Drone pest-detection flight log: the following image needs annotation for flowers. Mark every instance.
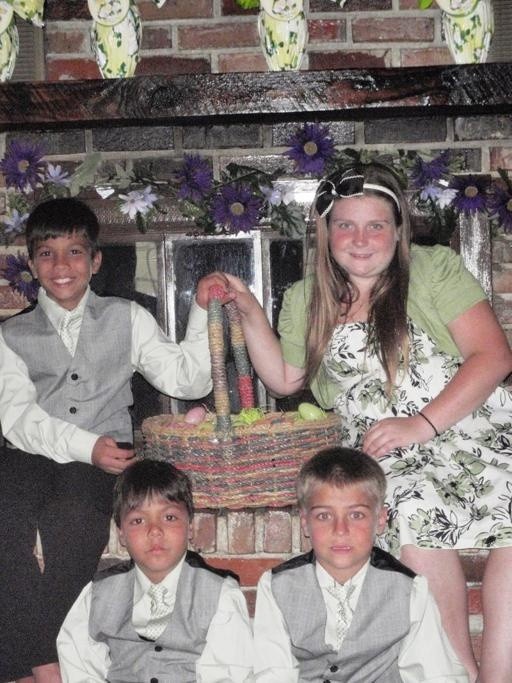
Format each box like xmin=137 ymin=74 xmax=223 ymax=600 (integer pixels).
xmin=0 ymin=120 xmax=512 ymax=303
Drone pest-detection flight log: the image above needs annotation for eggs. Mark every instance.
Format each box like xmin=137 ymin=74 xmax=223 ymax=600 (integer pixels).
xmin=298 ymin=401 xmax=324 ymax=421
xmin=183 ymin=405 xmax=207 ymax=427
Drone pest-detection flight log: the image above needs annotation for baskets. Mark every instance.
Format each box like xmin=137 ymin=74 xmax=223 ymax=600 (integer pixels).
xmin=140 ymin=284 xmax=343 ymax=513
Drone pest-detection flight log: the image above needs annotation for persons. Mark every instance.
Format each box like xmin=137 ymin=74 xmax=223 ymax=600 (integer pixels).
xmin=55 ymin=462 xmax=253 ymax=682
xmin=1 ymin=200 xmax=229 ymax=683
xmin=254 ymin=448 xmax=468 ymax=682
xmin=223 ymin=162 xmax=511 ymax=682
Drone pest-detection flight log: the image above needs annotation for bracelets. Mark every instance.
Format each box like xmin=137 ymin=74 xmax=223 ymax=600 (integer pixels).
xmin=418 ymin=412 xmax=439 ymax=436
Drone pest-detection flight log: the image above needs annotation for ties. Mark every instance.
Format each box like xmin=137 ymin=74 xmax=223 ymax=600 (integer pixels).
xmin=57 ymin=312 xmax=82 ymax=357
xmin=142 ymin=585 xmax=174 ymax=642
xmin=325 ymin=583 xmax=358 ymax=644
